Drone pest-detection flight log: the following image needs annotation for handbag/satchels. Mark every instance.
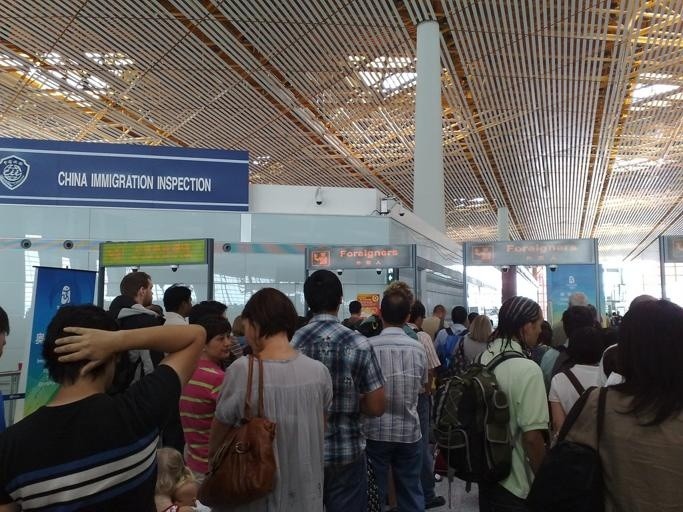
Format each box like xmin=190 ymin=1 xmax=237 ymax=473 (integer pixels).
xmin=524 ymin=386 xmax=609 ymax=511
xmin=197 ymin=353 xmax=279 ymax=511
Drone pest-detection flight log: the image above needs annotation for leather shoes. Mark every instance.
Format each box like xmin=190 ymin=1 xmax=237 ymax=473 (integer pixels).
xmin=426 ymin=495 xmax=446 ymax=508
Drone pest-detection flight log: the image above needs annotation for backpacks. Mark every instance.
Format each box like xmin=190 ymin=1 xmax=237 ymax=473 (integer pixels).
xmin=436 ymin=328 xmax=470 ymax=391
xmin=444 ymin=327 xmax=468 ymax=360
xmin=430 ymin=350 xmax=527 ymax=493
xmin=433 ymin=318 xmax=446 ymax=342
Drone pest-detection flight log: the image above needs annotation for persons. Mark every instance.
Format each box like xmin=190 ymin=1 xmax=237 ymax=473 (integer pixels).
xmin=405 ymin=299 xmax=445 ymax=509
xmin=420 ymin=305 xmax=447 ymax=344
xmin=178 ymin=314 xmax=232 ymax=484
xmin=340 ymin=301 xmax=367 ymax=331
xmin=526 ymin=321 xmax=552 ymax=366
xmin=205 ymin=287 xmax=333 ymax=512
xmin=467 ymin=313 xmax=478 ymax=325
xmin=0 ymin=303 xmax=206 ymax=512
xmin=188 ymin=300 xmax=227 ymax=325
xmin=360 ymin=289 xmax=428 ymax=512
xmin=171 ymin=479 xmax=211 ymax=512
xmin=231 ymin=315 xmax=248 ymax=350
xmin=154 ymin=448 xmax=193 ymax=512
xmin=469 ymin=296 xmax=548 ymax=512
xmin=548 ymin=320 xmax=607 ymax=435
xmin=288 ymin=269 xmax=386 ymax=512
xmin=596 ymin=344 xmax=625 ymax=387
xmin=550 ymin=292 xmax=587 ymax=348
xmin=539 ymin=305 xmax=606 ymax=399
xmin=450 ymin=315 xmax=493 ymax=365
xmin=161 ymin=283 xmax=192 ymax=359
xmin=104 ymin=272 xmax=165 ymax=393
xmin=433 ymin=306 xmax=471 ymax=351
xmin=0 ymin=307 xmax=9 ymax=431
xmin=382 ymin=280 xmax=418 ymax=341
xmin=550 ymin=299 xmax=682 ymax=512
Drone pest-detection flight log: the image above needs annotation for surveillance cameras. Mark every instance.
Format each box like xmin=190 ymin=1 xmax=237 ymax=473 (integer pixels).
xmin=131 ymin=266 xmax=140 ymax=272
xmin=502 ymin=265 xmax=509 ymax=272
xmin=550 ymin=264 xmax=557 ymax=271
xmin=397 ymin=206 xmax=404 ymax=216
xmin=171 ymin=265 xmax=179 ymax=271
xmin=375 ymin=268 xmax=381 ymax=274
xmin=337 ymin=269 xmax=343 ymax=275
xmin=316 ymin=196 xmax=322 ymax=204
